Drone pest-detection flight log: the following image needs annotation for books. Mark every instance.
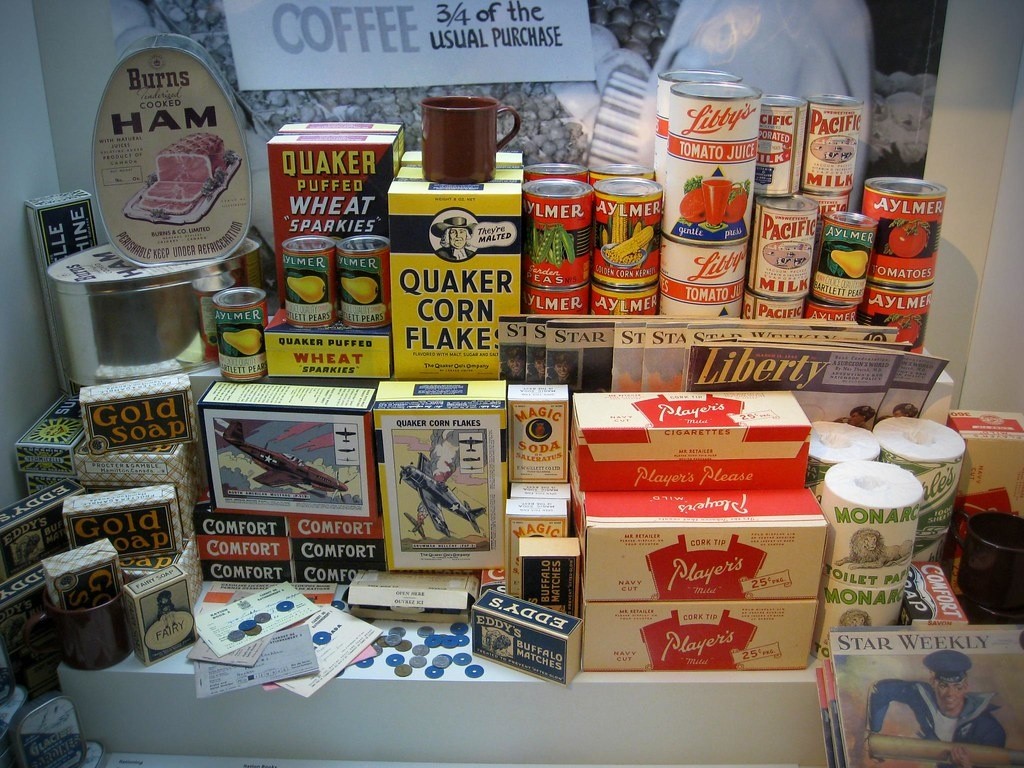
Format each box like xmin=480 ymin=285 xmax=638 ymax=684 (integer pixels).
xmin=499 ymin=315 xmax=949 ymax=429
xmin=814 ymin=625 xmax=1024 ymax=768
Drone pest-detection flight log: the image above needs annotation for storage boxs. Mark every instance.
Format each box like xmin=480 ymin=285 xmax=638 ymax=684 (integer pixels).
xmin=264 ymin=122 xmax=525 ymax=381
xmin=27 ymin=188 xmax=98 ymax=396
xmin=901 ymin=561 xmax=969 ymax=625
xmin=946 ymin=409 xmax=1024 ymax=598
xmin=571 ymin=391 xmax=831 ymax=672
xmin=50 ymin=34 xmax=260 ymax=388
xmin=0 ymin=374 xmax=203 ymax=767
xmin=193 ymin=373 xmax=583 ymax=686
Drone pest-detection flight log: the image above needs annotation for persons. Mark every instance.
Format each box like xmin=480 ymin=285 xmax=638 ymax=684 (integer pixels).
xmin=875 ymin=402 xmax=917 ymax=424
xmin=834 ymin=405 xmax=875 ymax=428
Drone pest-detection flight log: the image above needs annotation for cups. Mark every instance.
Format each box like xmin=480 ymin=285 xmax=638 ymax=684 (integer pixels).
xmin=950 ymin=508 xmax=1024 ymax=613
xmin=23 ymin=587 xmax=134 ymax=670
xmin=418 ymin=96 xmax=520 ymax=184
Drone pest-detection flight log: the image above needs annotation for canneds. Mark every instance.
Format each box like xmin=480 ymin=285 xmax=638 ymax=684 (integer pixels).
xmin=281 ymin=236 xmax=338 ymax=328
xmin=192 ymin=277 xmax=236 ymax=361
xmin=335 ymin=234 xmax=393 ymax=329
xmin=522 ymin=71 xmax=951 ymax=355
xmin=211 ymin=287 xmax=269 ymax=382
